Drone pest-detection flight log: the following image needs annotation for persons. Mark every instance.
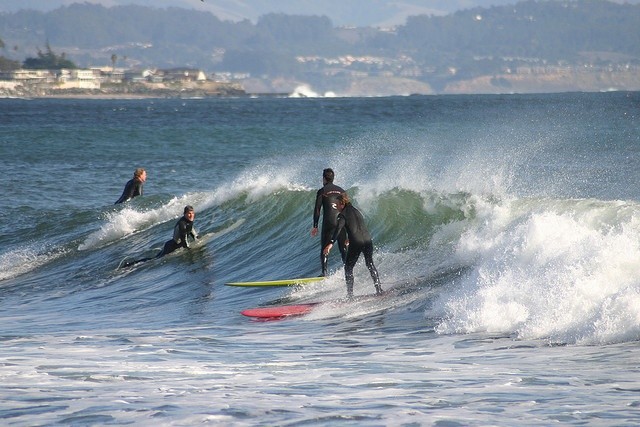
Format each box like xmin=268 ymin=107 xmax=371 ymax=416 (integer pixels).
xmin=310 ymin=168 xmax=348 ymax=277
xmin=323 ymin=193 xmax=383 ymax=299
xmin=123 ymin=205 xmax=197 ymax=268
xmin=114 ymin=168 xmax=146 ymax=205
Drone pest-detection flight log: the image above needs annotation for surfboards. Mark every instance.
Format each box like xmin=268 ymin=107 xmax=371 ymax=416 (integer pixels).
xmin=224 ymin=276 xmax=332 ymax=286
xmin=241 ymin=292 xmax=392 ymax=317
